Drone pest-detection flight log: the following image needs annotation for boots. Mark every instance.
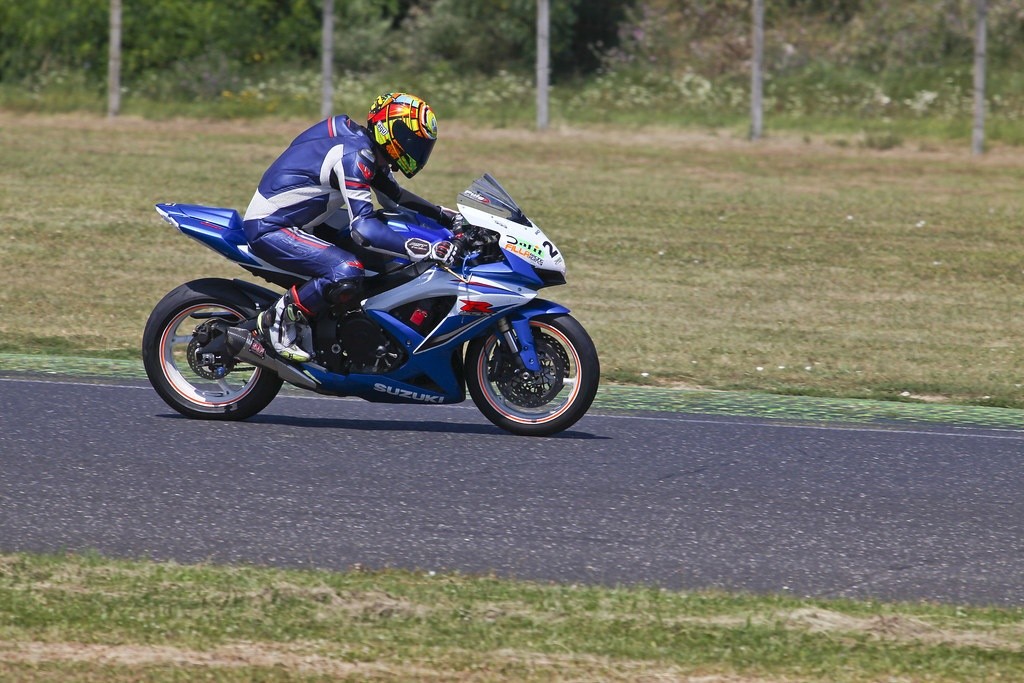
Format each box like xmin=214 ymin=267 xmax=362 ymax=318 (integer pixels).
xmin=256 ymin=283 xmax=318 ymax=363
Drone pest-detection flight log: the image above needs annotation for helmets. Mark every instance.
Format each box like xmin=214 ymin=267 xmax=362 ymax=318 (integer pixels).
xmin=368 ymin=93 xmax=438 ymax=179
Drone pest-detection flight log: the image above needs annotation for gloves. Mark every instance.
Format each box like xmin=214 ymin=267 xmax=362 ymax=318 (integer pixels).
xmin=405 ymin=236 xmax=460 ymax=267
xmin=437 ymin=206 xmax=470 ymax=235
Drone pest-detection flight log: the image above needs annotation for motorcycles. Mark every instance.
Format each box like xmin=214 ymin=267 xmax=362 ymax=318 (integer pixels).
xmin=142 ymin=172 xmax=601 ymax=437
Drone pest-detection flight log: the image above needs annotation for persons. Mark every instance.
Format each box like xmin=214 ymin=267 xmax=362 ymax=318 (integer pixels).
xmin=240 ymin=92 xmax=457 ymax=364
